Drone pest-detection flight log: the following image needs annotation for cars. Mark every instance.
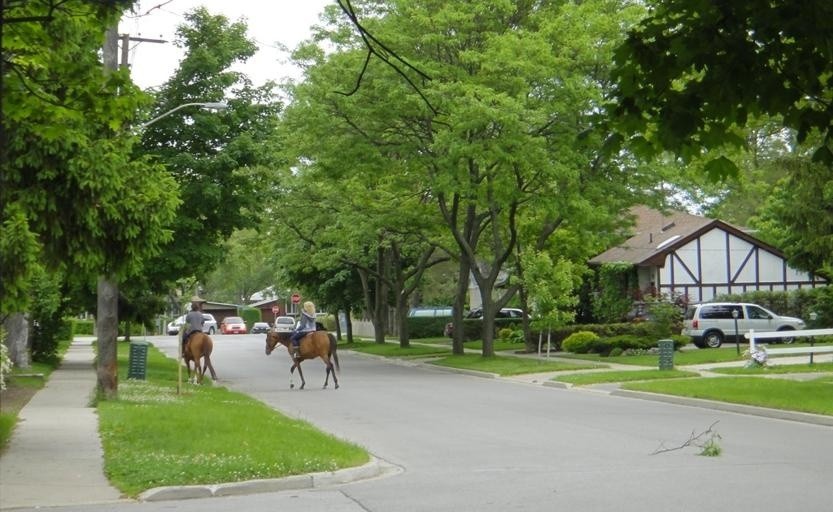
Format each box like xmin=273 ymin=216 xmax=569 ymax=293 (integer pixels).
xmin=167 ymin=313 xmax=296 ymax=335
xmin=445 ymin=307 xmax=533 ymax=342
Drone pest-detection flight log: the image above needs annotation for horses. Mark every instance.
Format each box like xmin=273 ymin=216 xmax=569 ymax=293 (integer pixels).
xmin=266 ymin=328 xmax=339 ymax=389
xmin=184 ymin=331 xmax=218 ymax=385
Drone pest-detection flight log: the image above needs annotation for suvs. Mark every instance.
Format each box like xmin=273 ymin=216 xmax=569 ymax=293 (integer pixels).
xmin=682 ymin=303 xmax=807 ymax=349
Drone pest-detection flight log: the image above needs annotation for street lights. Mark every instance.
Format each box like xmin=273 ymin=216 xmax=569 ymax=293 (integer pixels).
xmin=130 ymin=103 xmax=229 ymax=131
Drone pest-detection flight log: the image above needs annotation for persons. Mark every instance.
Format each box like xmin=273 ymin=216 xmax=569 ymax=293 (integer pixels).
xmin=289 ymin=300 xmax=316 ymax=359
xmin=181 ymin=303 xmax=204 ymax=358
